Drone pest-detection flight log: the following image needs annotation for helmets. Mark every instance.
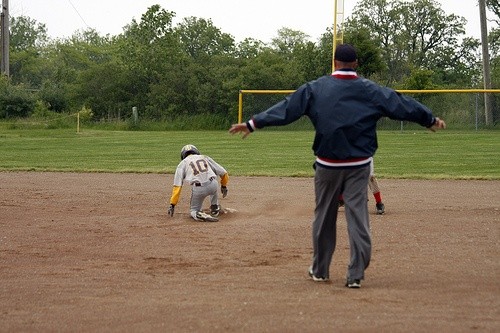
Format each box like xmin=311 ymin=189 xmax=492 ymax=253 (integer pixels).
xmin=181 ymin=144 xmax=200 ymax=159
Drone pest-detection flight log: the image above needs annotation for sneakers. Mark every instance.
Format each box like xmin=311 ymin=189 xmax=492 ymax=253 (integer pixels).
xmin=307 ymin=264 xmax=328 ymax=281
xmin=344 ymin=279 xmax=361 ymax=288
xmin=211 ymin=203 xmax=220 ymax=217
xmin=196 ymin=211 xmax=217 ymax=222
xmin=375 ymin=202 xmax=385 ymax=214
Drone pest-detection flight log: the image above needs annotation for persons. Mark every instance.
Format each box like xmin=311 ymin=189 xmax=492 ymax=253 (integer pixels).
xmin=229 ymin=45 xmax=446 ymax=289
xmin=368 ymin=170 xmax=385 ymax=214
xmin=167 ymin=144 xmax=229 ymax=222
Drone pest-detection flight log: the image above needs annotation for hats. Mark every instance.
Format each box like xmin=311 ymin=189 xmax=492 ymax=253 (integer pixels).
xmin=335 ymin=44 xmax=357 ymax=62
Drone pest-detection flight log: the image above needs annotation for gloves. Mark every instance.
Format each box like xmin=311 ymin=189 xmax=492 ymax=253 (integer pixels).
xmin=167 ymin=204 xmax=175 ymax=218
xmin=221 ymin=185 xmax=228 ymax=198
